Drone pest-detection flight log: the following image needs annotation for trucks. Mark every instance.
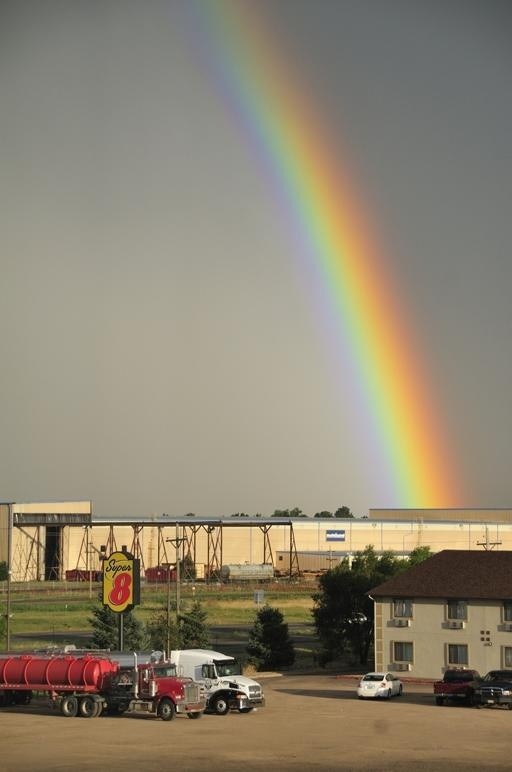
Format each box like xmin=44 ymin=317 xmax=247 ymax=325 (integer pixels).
xmin=142 ymin=566 xmax=182 ymax=583
xmin=64 ymin=568 xmax=103 ymax=582
xmin=26 ymin=643 xmax=265 ymax=715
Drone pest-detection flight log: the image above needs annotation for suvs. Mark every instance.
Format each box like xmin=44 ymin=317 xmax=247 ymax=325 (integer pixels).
xmin=474 ymin=670 xmax=512 ymax=711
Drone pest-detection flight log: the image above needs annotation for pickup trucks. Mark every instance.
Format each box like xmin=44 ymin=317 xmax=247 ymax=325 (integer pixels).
xmin=434 ymin=667 xmax=481 ymax=707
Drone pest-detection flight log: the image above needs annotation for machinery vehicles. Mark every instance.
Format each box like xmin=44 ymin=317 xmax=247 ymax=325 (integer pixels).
xmin=217 ymin=562 xmax=288 ymax=584
xmin=0 ymin=651 xmax=202 ymax=722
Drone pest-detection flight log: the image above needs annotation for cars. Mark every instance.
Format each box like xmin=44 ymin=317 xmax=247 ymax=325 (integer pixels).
xmin=343 ymin=610 xmax=368 ymax=623
xmin=351 ymin=671 xmax=406 ymax=700
